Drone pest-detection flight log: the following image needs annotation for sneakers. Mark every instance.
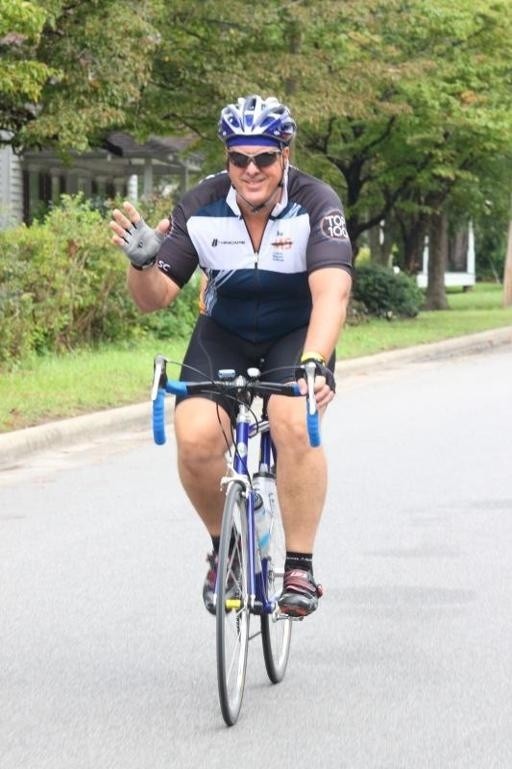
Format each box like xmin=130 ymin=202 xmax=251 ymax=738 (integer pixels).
xmin=202 ymin=548 xmax=241 ymax=617
xmin=277 ymin=564 xmax=325 ymax=618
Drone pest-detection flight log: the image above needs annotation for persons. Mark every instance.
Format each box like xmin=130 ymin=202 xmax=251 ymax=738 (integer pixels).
xmin=109 ymin=93 xmax=355 ymax=617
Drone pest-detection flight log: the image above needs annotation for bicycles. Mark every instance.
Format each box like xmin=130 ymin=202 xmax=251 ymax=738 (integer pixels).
xmin=142 ymin=354 xmax=333 ymax=730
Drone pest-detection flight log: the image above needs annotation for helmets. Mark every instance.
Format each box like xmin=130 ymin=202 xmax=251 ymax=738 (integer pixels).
xmin=215 ymin=93 xmax=297 ymax=146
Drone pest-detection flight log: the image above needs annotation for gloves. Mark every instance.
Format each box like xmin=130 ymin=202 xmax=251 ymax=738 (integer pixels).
xmin=116 ymin=215 xmax=167 ymax=270
xmin=294 ymin=358 xmax=337 ymax=394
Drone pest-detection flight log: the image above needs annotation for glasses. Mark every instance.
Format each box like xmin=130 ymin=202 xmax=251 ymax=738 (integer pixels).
xmin=225 ymin=148 xmax=283 ymax=169
